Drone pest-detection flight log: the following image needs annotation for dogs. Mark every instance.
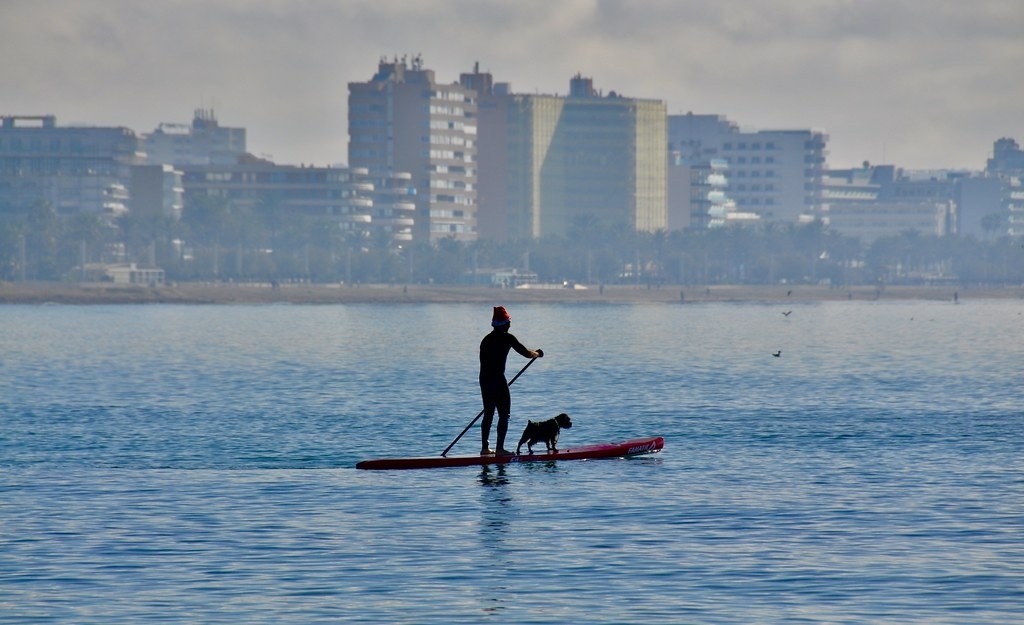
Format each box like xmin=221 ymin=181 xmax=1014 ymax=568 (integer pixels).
xmin=516 ymin=413 xmax=573 ymax=452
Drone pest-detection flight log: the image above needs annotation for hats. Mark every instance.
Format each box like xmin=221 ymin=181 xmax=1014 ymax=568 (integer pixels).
xmin=491 ymin=306 xmax=511 ymax=326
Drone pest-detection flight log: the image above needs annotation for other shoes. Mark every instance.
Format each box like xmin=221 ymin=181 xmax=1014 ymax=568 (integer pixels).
xmin=495 ymin=450 xmax=516 ymax=457
xmin=480 ymin=448 xmax=496 ymax=455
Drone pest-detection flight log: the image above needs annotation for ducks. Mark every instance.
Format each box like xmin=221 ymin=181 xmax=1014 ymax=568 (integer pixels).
xmin=772 ymin=351 xmax=781 ymax=358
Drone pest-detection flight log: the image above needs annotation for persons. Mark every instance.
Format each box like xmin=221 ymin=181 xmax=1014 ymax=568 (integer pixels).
xmin=479 ymin=305 xmax=544 ymax=455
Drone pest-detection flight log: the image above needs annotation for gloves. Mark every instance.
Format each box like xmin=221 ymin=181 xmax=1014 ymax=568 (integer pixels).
xmin=535 ymin=349 xmax=544 ymax=358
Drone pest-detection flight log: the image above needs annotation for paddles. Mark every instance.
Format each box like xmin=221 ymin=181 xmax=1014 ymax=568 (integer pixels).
xmin=439 ymin=356 xmax=537 ymax=457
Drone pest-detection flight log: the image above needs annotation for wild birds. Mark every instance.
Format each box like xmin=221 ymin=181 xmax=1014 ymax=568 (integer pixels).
xmin=782 ymin=311 xmax=793 ymax=315
